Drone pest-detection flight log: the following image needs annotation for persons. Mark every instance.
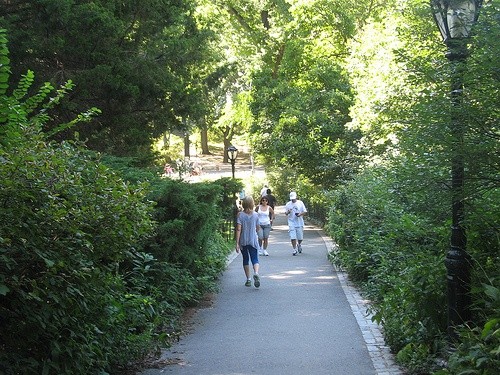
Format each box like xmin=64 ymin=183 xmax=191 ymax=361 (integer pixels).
xmin=239 ymin=188 xmax=245 ymax=208
xmin=235 ymin=197 xmax=261 ymax=288
xmin=285 ymin=192 xmax=307 ymax=255
xmin=254 ymin=184 xmax=276 ymax=256
xmin=164 ymin=159 xmax=203 ymax=177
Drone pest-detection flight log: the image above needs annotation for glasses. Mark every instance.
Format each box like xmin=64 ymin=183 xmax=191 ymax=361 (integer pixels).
xmin=262 ymin=200 xmax=267 ymax=202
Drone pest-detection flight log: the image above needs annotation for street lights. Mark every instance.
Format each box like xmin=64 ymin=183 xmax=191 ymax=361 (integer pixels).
xmin=226 ymin=145 xmax=239 ymax=227
xmin=430 ymin=1 xmax=488 ymax=344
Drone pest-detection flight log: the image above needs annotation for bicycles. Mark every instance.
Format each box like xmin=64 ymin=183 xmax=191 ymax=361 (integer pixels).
xmin=163 ymin=156 xmax=204 ymax=176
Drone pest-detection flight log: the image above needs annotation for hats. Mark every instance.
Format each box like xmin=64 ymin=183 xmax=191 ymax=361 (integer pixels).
xmin=290 ymin=192 xmax=296 ymax=199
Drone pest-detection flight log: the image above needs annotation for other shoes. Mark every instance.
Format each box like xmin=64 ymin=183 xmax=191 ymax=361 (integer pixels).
xmin=297 ymin=244 xmax=302 ymax=253
xmin=293 ymin=249 xmax=297 ymax=254
xmin=253 ymin=272 xmax=260 ymax=288
xmin=263 ymin=251 xmax=269 ymax=255
xmin=259 ymin=250 xmax=263 ymax=255
xmin=245 ymin=280 xmax=251 ymax=286
